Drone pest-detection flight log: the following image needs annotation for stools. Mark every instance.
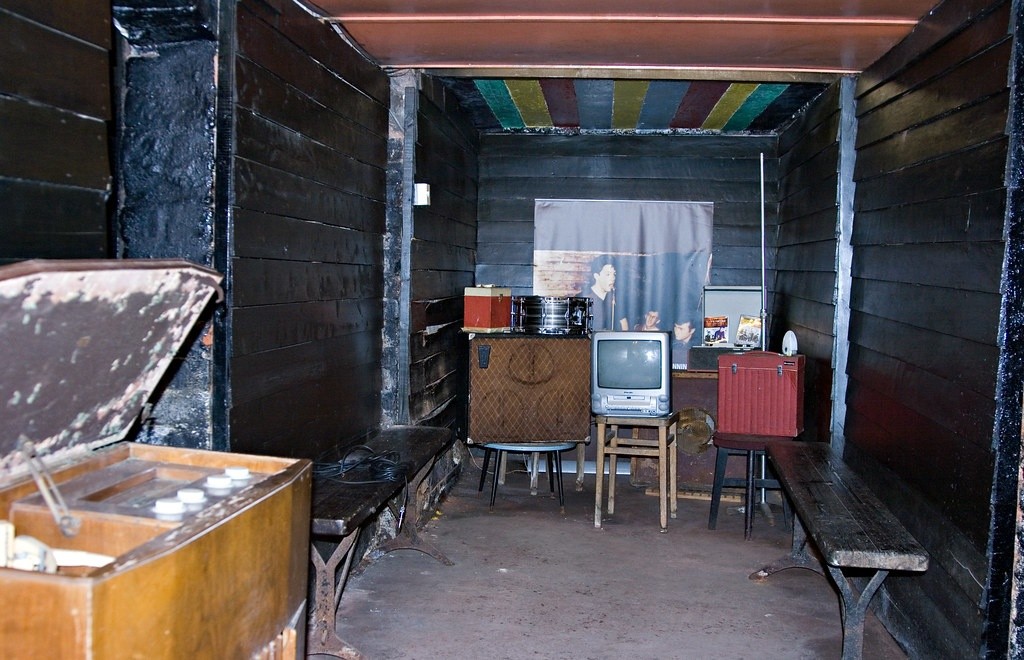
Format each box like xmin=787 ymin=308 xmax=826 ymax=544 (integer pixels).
xmin=708 ymin=435 xmax=790 ymax=540
xmin=594 ymin=412 xmax=679 ymax=530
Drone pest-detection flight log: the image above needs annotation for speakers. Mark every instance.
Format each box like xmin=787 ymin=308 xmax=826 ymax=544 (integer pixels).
xmin=467 ymin=332 xmax=590 ymax=445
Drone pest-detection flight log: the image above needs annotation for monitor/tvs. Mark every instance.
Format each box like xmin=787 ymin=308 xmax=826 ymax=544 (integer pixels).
xmin=591 ymin=330 xmax=671 ymax=418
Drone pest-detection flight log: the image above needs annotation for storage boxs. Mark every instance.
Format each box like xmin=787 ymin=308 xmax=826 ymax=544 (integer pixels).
xmin=711 ymin=354 xmax=804 ymax=436
xmin=461 ymin=287 xmax=514 ymax=334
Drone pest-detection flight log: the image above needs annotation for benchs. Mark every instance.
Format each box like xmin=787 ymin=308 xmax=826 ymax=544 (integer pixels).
xmin=748 ymin=435 xmax=932 ymax=659
xmin=304 ymin=423 xmax=462 ymax=660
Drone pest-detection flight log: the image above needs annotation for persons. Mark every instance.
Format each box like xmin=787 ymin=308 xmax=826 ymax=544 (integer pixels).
xmin=573 ymin=255 xmax=629 ymax=332
xmin=634 ymin=308 xmax=661 ymax=333
xmin=672 ymin=314 xmax=703 ymax=348
xmin=705 ymin=327 xmax=725 ymax=342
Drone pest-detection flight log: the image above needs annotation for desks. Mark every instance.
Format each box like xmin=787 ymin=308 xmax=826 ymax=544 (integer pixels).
xmin=476 ymin=440 xmax=576 ymax=511
